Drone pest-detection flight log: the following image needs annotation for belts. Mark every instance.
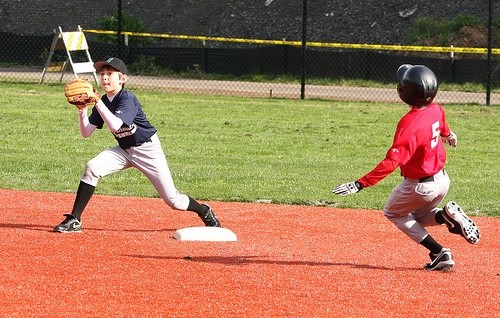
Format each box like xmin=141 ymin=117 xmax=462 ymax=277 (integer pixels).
xmin=419 ymin=168 xmax=445 ymax=183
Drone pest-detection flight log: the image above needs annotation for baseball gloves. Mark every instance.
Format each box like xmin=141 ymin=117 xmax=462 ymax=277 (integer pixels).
xmin=64 ymin=80 xmax=103 ymax=106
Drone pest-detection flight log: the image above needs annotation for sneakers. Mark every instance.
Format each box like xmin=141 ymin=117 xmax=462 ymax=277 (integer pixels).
xmin=53 ymin=213 xmax=82 ymax=232
xmin=442 ymin=201 xmax=480 ymax=244
xmin=198 ymin=204 xmax=221 ymax=228
xmin=423 ymin=248 xmax=455 ymax=270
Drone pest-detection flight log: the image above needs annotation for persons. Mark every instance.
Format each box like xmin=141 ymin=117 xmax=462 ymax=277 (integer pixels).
xmin=54 ymin=57 xmax=221 ymax=233
xmin=333 ymin=61 xmax=481 ymax=271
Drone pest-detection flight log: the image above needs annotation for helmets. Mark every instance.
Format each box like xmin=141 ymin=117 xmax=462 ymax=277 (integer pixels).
xmin=396 ymin=63 xmax=438 ymax=106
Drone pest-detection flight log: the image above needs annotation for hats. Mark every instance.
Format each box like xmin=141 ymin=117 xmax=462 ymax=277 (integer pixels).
xmin=94 ymin=57 xmax=128 ymax=75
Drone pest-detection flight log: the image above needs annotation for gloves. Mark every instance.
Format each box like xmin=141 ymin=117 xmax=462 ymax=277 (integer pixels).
xmin=332 ymin=180 xmax=363 ymax=196
xmin=441 ymin=132 xmax=458 ymax=147
